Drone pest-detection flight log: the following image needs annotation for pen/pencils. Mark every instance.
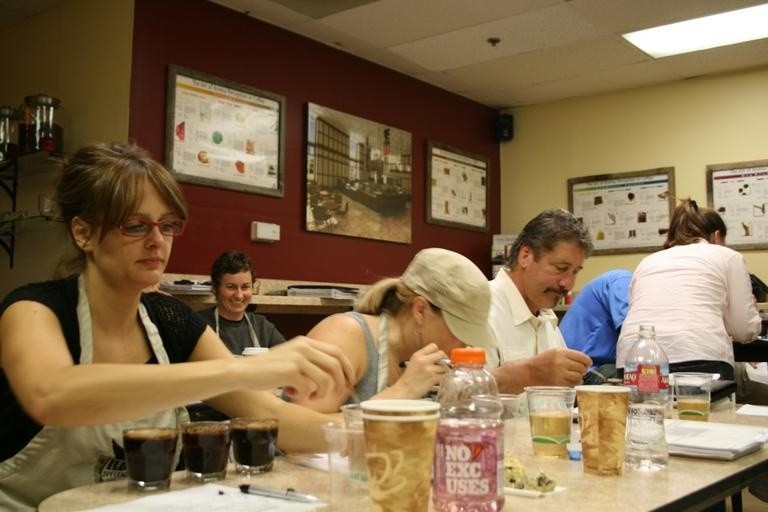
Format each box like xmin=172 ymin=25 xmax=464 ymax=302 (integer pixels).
xmin=399 ymin=359 xmax=452 ymax=367
xmin=238 ymin=484 xmax=317 ymax=503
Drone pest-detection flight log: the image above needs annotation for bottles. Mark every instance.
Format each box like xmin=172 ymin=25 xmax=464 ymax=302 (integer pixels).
xmin=625 ymin=324 xmax=669 ymax=473
xmin=431 ymin=347 xmax=506 ymax=511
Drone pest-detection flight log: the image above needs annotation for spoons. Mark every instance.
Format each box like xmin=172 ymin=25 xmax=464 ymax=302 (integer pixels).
xmin=588 ymin=367 xmax=623 ymax=383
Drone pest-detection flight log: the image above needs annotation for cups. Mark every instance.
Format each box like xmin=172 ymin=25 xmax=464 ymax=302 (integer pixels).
xmin=471 ymin=392 xmax=521 ymax=456
xmin=228 ymin=417 xmax=281 ymax=474
xmin=241 ymin=347 xmax=269 ymax=357
xmin=180 ymin=421 xmax=229 ymax=480
xmin=124 ymin=427 xmax=176 ymax=490
xmin=672 ymin=371 xmax=714 ymax=422
xmin=321 ymin=397 xmax=442 ymax=511
xmin=575 ymin=384 xmax=631 ymax=476
xmin=523 ymin=385 xmax=577 ymax=458
xmin=564 ymin=290 xmax=571 ymax=304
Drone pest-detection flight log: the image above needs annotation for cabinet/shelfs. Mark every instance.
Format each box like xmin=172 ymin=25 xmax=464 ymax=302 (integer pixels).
xmin=1 ymin=145 xmax=68 ymax=269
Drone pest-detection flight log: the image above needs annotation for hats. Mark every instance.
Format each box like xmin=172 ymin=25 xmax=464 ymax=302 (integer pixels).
xmin=400 ymin=248 xmax=498 ymax=348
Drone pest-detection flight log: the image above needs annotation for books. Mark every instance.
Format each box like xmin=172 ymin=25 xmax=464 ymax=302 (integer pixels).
xmin=667 ymin=418 xmax=761 ymax=460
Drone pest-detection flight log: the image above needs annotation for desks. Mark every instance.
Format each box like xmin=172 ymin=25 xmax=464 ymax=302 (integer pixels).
xmin=34 ymin=400 xmax=768 ymax=511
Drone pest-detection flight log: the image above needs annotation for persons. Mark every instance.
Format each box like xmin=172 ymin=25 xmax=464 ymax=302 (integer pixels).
xmin=557 ymin=269 xmax=633 ymax=384
xmin=0 ymin=141 xmax=356 ymax=512
xmin=195 ymin=250 xmax=285 ymax=354
xmin=280 ymin=248 xmax=494 ymax=413
xmin=483 ymin=208 xmax=593 ymax=394
xmin=615 ymin=198 xmax=761 ymax=380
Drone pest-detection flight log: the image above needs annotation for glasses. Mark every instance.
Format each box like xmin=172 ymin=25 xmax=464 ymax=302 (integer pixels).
xmin=87 ymin=214 xmax=187 ymax=237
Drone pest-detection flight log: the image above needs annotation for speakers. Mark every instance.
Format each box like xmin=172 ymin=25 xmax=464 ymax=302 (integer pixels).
xmin=495 ymin=115 xmax=513 ymax=140
